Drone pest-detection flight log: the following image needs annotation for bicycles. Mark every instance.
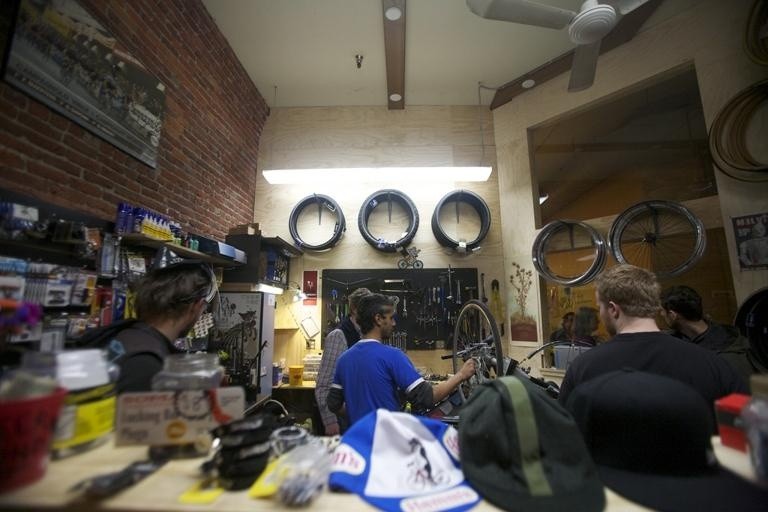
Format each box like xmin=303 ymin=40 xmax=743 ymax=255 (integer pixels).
xmin=443 ymin=299 xmax=597 ymax=404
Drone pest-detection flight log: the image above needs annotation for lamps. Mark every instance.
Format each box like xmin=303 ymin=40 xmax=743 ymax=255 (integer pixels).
xmin=260 ymin=160 xmax=491 ymax=188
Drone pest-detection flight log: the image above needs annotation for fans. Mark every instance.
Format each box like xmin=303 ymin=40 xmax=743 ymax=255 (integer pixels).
xmin=466 ymin=0 xmax=656 ymax=93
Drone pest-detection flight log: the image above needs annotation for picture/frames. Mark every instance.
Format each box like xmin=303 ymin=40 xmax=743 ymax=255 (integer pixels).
xmin=503 ymin=253 xmax=544 ymax=348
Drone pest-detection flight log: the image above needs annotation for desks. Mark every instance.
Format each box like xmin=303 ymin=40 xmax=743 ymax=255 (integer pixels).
xmin=274 ymin=374 xmax=317 ymax=394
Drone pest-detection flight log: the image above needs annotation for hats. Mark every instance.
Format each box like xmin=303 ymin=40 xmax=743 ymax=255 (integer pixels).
xmin=561 ymin=312 xmax=574 ymax=321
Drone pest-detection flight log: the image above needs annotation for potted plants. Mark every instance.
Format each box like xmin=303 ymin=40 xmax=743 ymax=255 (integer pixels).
xmin=507 ymin=259 xmax=540 ymax=344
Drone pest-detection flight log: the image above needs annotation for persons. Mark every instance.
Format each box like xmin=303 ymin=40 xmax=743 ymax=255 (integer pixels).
xmin=558 ymin=263 xmax=747 ymax=418
xmin=312 ymin=287 xmax=376 ymax=432
xmin=660 ymin=284 xmax=752 ymax=397
xmin=329 ymin=292 xmax=476 ymax=426
xmin=90 ymin=264 xmax=216 ymax=391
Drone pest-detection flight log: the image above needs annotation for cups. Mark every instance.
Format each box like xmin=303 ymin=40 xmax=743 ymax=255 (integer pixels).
xmin=270 ymin=425 xmax=308 ymax=456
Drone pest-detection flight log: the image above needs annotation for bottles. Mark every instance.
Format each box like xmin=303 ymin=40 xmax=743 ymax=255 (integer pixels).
xmin=741 ymin=373 xmax=768 ymax=493
xmin=148 ymin=353 xmax=223 ymax=461
xmin=288 ymin=366 xmax=304 ymax=386
xmin=273 ymin=362 xmax=282 ymax=385
